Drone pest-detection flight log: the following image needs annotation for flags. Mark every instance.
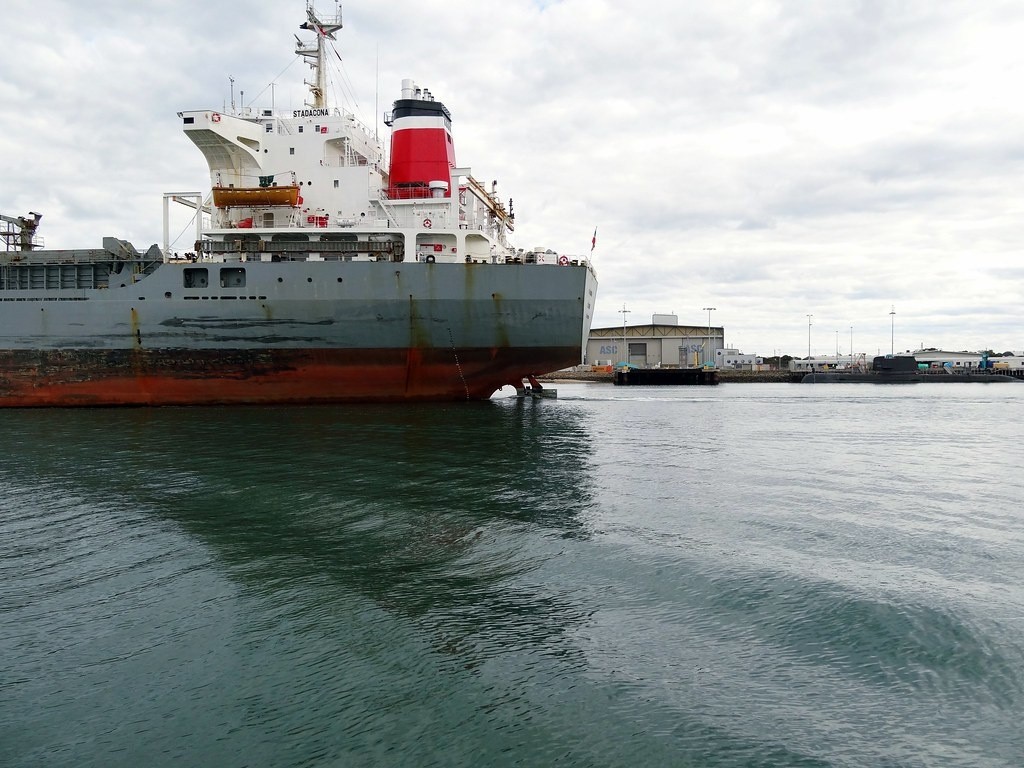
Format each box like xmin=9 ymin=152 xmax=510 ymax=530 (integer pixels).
xmin=592 ymin=229 xmax=597 ymax=249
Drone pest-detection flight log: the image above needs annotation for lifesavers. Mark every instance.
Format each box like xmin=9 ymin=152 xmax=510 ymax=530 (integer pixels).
xmin=423 ymin=217 xmax=432 ymax=229
xmin=560 ymin=255 xmax=569 ymax=265
xmin=425 ymin=254 xmax=436 ymax=265
xmin=211 ymin=112 xmax=220 ymax=122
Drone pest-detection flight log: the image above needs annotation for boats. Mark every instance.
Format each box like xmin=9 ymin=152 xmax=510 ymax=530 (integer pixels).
xmin=0 ymin=0 xmax=597 ymax=407
xmin=612 ymin=367 xmax=720 ymax=384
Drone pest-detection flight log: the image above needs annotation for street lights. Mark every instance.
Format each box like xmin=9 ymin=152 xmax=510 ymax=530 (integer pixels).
xmin=850 ymin=327 xmax=853 ymax=374
xmin=889 ymin=312 xmax=896 ymax=357
xmin=806 ymin=315 xmax=813 ymax=370
xmin=618 ymin=311 xmax=631 ymax=366
xmin=703 ymin=308 xmax=717 ymax=362
